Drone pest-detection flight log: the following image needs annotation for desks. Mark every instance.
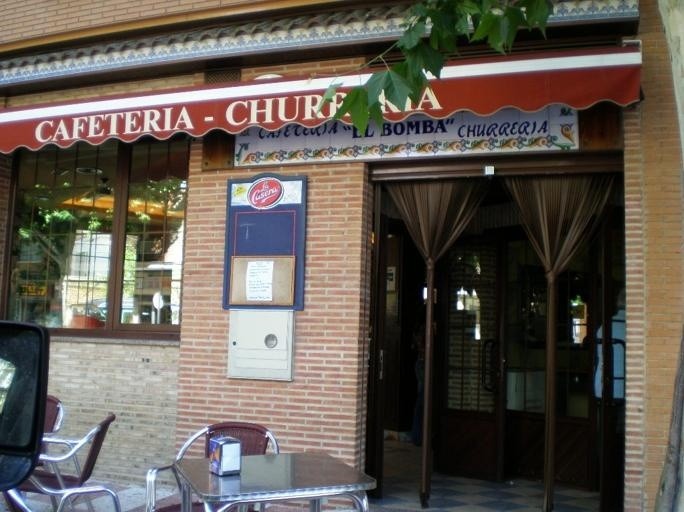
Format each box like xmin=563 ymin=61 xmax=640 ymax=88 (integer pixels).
xmin=174 ymin=452 xmax=377 ymax=512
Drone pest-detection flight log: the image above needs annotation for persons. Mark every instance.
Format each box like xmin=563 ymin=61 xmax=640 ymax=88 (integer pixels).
xmin=590 ymin=287 xmax=624 ymax=511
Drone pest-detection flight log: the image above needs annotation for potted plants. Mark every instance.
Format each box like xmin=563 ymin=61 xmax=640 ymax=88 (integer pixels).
xmin=71 ymin=308 xmax=105 ymax=328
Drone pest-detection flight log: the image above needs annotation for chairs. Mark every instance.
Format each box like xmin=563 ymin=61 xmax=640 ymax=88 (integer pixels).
xmin=3 ymin=395 xmax=120 ymax=512
xmin=146 ymin=423 xmax=279 ymax=512
